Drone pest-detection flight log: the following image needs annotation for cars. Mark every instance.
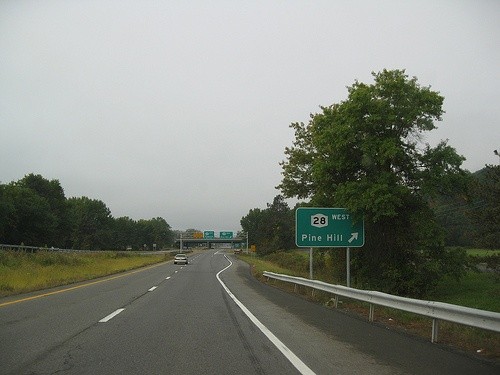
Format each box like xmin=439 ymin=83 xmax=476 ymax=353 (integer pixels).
xmin=174 ymin=253 xmax=188 ymax=264
